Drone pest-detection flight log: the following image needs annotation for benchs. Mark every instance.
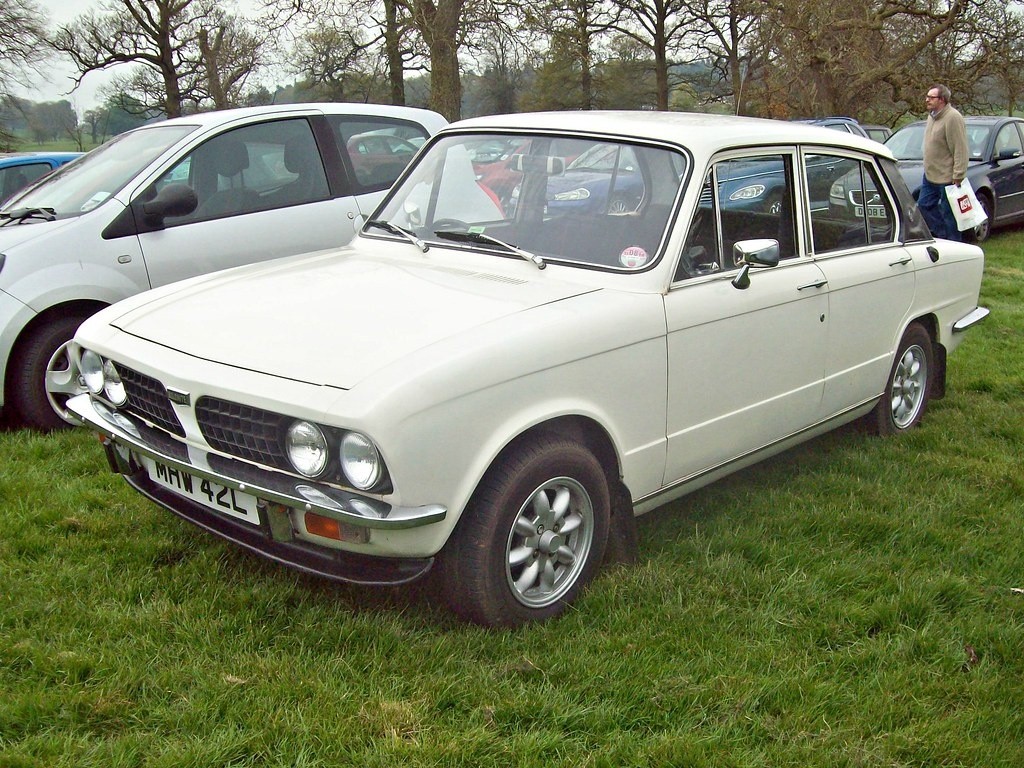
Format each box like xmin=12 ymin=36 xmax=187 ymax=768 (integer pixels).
xmin=693 ymin=207 xmax=847 ymax=266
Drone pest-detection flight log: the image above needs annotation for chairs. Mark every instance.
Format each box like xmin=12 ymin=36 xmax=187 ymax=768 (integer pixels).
xmin=194 ymin=142 xmax=258 ymax=218
xmin=277 ymin=136 xmax=327 ymax=202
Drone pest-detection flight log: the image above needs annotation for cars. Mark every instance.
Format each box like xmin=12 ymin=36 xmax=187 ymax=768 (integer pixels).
xmin=0 ymin=102 xmax=508 ymax=439
xmin=0 ymin=149 xmax=92 ymax=210
xmin=829 ymin=114 xmax=1024 ymax=243
xmin=159 ymin=115 xmax=896 ymax=219
xmin=61 ymin=108 xmax=992 ymax=633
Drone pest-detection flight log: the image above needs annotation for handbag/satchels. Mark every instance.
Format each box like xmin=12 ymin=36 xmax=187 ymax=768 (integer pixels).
xmin=944 ymin=177 xmax=988 ymax=231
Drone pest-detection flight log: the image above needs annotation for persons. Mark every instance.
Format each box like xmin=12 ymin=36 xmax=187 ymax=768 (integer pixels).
xmin=917 ymin=83 xmax=969 ymax=241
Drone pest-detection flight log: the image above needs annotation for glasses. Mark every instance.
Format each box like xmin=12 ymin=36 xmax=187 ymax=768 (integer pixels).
xmin=926 ymin=95 xmax=940 ymax=101
